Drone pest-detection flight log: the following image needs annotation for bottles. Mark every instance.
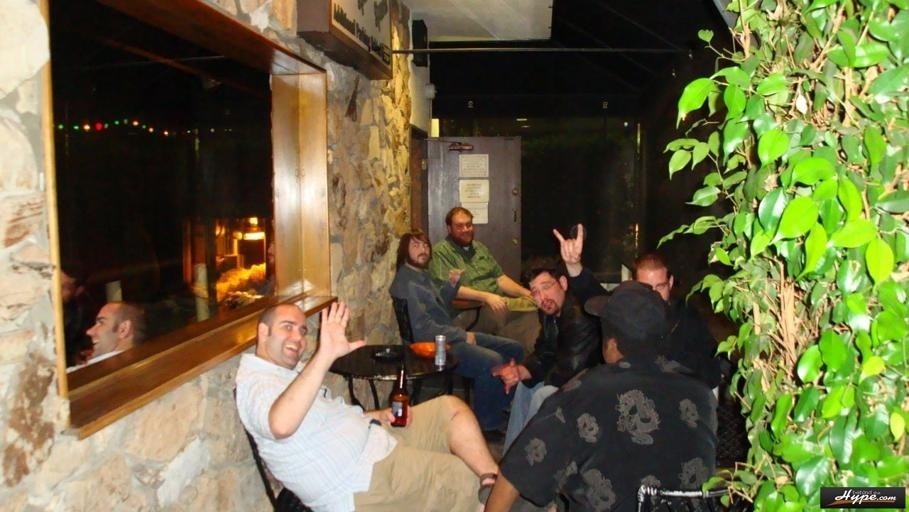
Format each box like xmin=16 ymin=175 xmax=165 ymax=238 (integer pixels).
xmin=434 ymin=334 xmax=446 ymax=369
xmin=387 ymin=363 xmax=408 ymax=428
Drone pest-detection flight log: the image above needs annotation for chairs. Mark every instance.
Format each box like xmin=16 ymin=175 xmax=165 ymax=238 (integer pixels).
xmin=226 ymin=393 xmax=314 ymax=511
xmin=393 ymin=298 xmax=414 ymax=342
xmin=636 ymin=484 xmax=758 ymax=512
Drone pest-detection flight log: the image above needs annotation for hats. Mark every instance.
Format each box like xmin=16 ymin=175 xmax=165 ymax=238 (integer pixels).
xmin=583 ymin=280 xmax=669 ymax=343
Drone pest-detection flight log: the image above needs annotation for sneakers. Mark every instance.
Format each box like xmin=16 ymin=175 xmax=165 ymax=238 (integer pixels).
xmin=482 ymin=409 xmax=512 ymax=445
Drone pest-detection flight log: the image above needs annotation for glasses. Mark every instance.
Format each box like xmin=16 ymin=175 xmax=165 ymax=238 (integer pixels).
xmin=529 ymin=280 xmax=559 ymax=298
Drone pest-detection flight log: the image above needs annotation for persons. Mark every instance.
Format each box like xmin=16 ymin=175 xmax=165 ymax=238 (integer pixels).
xmin=86 ymin=301 xmax=149 ymax=368
xmin=423 ymin=207 xmax=533 ymax=336
xmin=483 ymin=278 xmax=720 ymax=512
xmin=61 ymin=262 xmax=89 ymax=308
xmin=387 ymin=229 xmax=526 ymax=445
xmin=551 ymin=222 xmax=720 ymax=393
xmin=233 ymin=301 xmax=546 ymax=512
xmin=491 ymin=265 xmax=604 ymax=460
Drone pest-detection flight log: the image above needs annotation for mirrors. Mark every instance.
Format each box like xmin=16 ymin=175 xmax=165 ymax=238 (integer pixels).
xmin=40 ymin=0 xmax=340 ymax=438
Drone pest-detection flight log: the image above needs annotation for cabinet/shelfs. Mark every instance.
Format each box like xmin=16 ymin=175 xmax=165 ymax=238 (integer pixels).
xmin=452 ymin=298 xmax=487 ymax=335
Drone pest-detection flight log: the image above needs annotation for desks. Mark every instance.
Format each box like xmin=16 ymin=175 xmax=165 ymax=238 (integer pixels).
xmin=332 ymin=342 xmax=456 ymax=409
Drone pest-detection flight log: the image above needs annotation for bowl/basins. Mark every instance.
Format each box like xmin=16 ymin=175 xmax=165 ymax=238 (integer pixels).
xmin=369 ymin=348 xmax=407 ymax=363
xmin=409 ymin=341 xmax=452 ymax=359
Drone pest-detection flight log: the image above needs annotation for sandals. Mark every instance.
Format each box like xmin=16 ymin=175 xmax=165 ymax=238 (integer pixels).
xmin=478 ymin=471 xmax=561 ymax=512
